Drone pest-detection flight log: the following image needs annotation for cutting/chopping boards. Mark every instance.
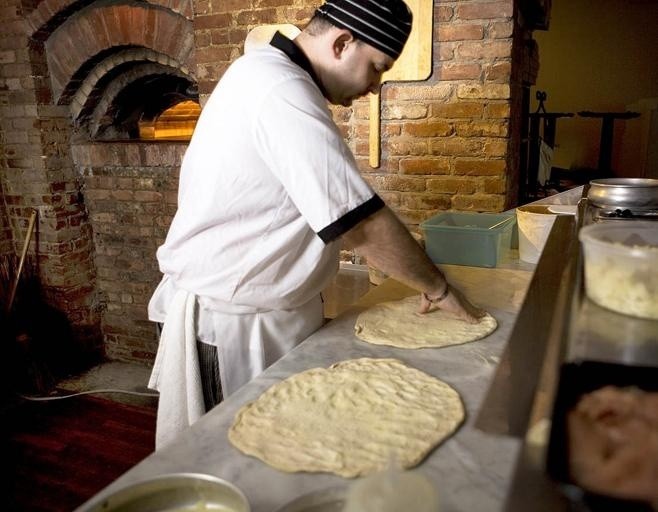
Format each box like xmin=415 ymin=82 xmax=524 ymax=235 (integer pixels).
xmin=367 ymin=1 xmax=433 ymax=169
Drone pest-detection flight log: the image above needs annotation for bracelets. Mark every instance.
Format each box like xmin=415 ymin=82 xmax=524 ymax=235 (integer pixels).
xmin=424 ymin=279 xmax=449 ymax=304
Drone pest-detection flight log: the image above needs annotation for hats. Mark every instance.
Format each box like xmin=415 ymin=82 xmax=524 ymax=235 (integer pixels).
xmin=315 ymin=0 xmax=413 ymax=60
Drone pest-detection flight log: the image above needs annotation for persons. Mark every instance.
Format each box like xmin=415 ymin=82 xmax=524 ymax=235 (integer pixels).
xmin=148 ymin=1 xmax=486 ymax=453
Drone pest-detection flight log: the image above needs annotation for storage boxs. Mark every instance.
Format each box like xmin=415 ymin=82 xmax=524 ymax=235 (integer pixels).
xmin=420 ymin=210 xmax=518 ymax=267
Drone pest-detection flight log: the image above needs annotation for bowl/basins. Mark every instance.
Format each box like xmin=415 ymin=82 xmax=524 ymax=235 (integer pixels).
xmin=577 ymin=219 xmax=658 ymax=319
xmin=586 ymin=178 xmax=658 ymax=211
xmin=82 ymin=473 xmax=249 ymax=512
